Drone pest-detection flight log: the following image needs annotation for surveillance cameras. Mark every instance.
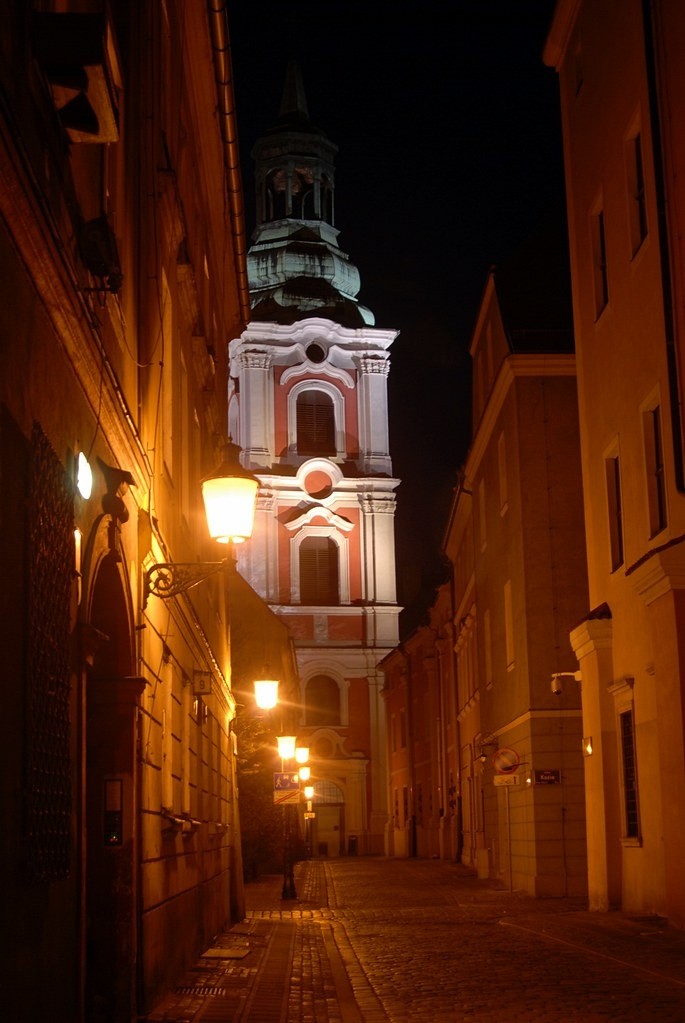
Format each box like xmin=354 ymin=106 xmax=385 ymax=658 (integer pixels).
xmin=551 ymin=679 xmax=562 ymax=695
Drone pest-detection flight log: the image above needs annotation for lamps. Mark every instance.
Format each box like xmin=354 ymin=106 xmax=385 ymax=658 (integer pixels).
xmin=229 ymin=666 xmax=279 ymax=736
xmin=143 ymin=433 xmax=261 ymax=607
xmin=480 ymin=743 xmax=497 ymax=762
